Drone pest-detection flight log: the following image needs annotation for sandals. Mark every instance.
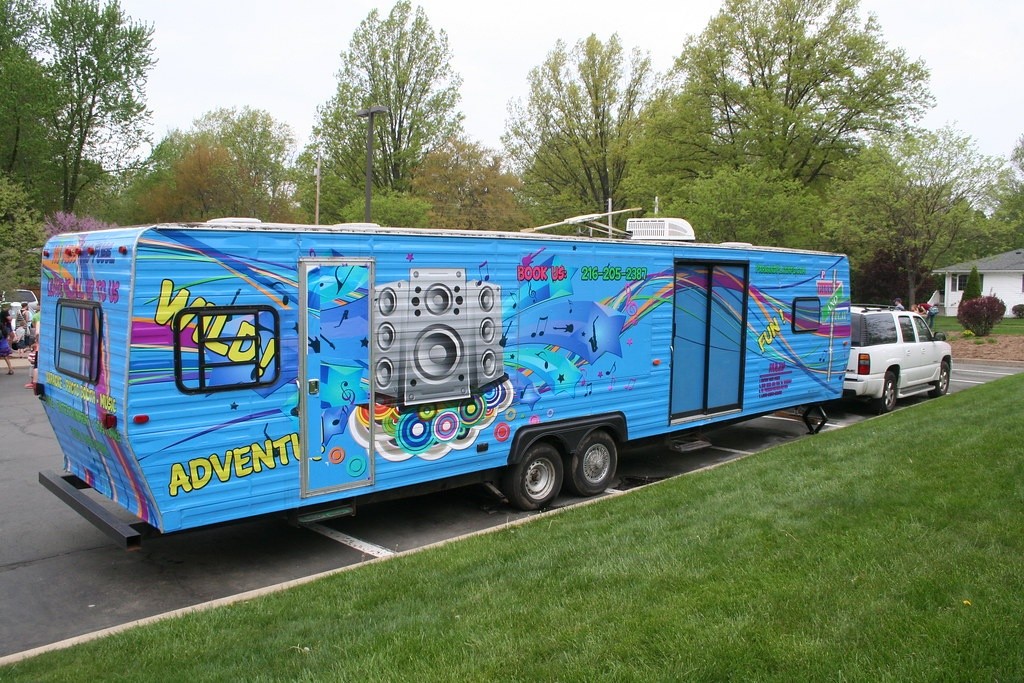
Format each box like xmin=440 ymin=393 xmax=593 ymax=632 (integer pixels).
xmin=7 ymin=368 xmax=13 ymax=374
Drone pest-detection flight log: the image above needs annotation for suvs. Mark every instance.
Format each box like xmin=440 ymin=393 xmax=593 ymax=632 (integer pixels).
xmin=839 ymin=303 xmax=954 ymax=414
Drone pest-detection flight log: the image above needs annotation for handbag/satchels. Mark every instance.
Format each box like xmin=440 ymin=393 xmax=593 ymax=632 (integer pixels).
xmin=926 ymin=306 xmax=939 ymax=315
xmin=26 ymin=324 xmax=29 ymax=336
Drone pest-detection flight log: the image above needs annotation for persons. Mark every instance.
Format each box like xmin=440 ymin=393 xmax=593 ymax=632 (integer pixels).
xmin=893 ymin=298 xmax=905 ymax=311
xmin=15 ymin=302 xmax=30 ymax=352
xmin=25 ymin=343 xmax=37 ymax=389
xmin=0 ymin=303 xmax=15 ymax=349
xmin=0 ymin=317 xmax=14 ymax=375
xmin=911 ymin=304 xmax=919 ymax=316
xmin=15 ymin=321 xmax=26 ymax=358
xmin=29 ymin=303 xmax=40 ymax=350
xmin=917 ymin=303 xmax=936 ymax=329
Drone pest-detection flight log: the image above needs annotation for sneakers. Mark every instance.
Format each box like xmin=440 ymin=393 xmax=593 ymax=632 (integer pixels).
xmin=24 ymin=382 xmax=34 ymax=388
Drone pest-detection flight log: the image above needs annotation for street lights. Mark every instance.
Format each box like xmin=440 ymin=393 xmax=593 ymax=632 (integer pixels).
xmin=356 ymin=106 xmax=388 ymax=223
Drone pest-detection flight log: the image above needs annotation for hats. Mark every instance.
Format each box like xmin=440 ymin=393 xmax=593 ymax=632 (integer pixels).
xmin=893 ymin=296 xmax=902 ymax=302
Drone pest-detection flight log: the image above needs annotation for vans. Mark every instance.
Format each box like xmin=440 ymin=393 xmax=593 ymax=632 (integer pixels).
xmin=0 ymin=289 xmax=39 ymax=331
